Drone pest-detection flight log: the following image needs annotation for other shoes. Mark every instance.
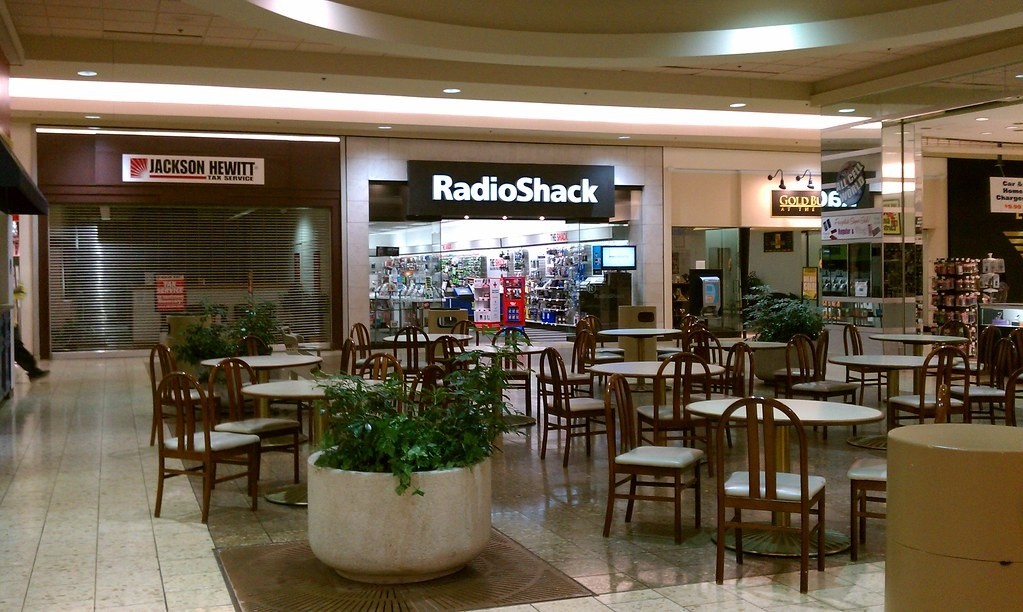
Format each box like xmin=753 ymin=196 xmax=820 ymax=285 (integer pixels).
xmin=26 ymin=368 xmax=50 ymax=382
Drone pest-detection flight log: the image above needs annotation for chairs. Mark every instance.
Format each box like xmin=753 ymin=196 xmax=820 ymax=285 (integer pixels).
xmin=149 ymin=313 xmax=1023 ymax=594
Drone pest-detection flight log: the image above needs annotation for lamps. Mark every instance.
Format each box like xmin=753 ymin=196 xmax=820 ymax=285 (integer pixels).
xmin=768 ymin=169 xmax=786 ymax=189
xmin=796 ymin=169 xmax=815 ymax=189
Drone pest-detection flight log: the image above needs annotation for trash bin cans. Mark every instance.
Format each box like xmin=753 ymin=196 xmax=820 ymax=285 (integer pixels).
xmin=884 ymin=424 xmax=1023 ymax=611
xmin=167 ymin=311 xmax=212 ymax=388
xmin=425 ymin=307 xmax=469 ymax=370
xmin=618 ymin=305 xmax=657 ymax=362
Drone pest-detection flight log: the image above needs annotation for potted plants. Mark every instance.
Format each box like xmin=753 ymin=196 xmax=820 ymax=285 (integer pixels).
xmin=741 ymin=285 xmax=827 ymax=386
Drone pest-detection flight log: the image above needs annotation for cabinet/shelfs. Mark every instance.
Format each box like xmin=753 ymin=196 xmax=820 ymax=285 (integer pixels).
xmin=821 ymin=243 xmax=1005 ymax=358
xmin=369 ymin=245 xmax=633 ymax=343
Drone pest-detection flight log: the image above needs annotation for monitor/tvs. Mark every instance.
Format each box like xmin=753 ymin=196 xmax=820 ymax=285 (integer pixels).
xmin=601 ymin=246 xmax=637 ymax=270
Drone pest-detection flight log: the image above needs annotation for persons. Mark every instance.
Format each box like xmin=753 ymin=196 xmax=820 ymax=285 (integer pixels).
xmin=12 ymin=327 xmax=51 ymax=384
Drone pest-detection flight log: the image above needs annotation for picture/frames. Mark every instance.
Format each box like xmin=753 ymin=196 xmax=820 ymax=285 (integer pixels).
xmin=882 ymin=198 xmax=902 ymax=236
xmin=763 ymin=230 xmax=794 ymax=253
